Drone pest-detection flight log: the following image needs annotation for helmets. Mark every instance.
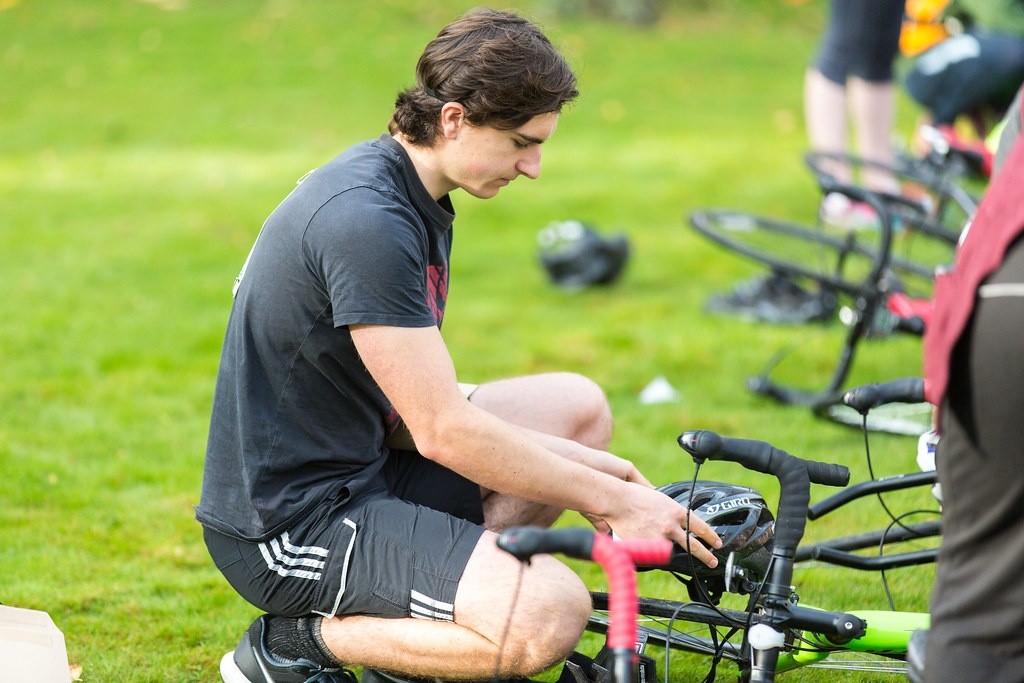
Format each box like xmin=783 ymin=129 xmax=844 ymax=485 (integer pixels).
xmin=648 ymin=479 xmax=775 ymax=602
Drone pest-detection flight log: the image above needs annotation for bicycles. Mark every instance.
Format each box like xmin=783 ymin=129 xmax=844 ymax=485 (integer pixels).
xmin=366 ymin=148 xmax=981 ymax=683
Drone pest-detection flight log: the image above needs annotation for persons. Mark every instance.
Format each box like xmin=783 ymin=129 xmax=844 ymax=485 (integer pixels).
xmin=802 ymin=0 xmax=1024 ymax=683
xmin=194 ymin=9 xmax=723 ymax=683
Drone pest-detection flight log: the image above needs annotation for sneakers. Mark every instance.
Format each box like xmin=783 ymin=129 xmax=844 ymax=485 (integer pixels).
xmin=361 ymin=667 xmax=422 ymax=683
xmin=220 ymin=614 xmax=357 ymax=683
